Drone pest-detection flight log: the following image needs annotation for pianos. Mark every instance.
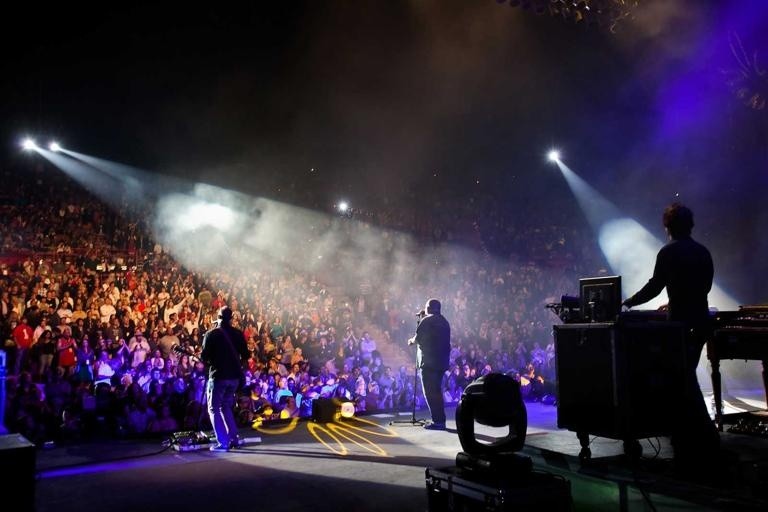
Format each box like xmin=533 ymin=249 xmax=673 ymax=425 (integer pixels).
xmin=557 ymin=306 xmax=740 ymax=325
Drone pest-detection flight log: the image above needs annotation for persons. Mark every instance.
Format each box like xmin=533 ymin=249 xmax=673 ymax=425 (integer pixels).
xmin=231 ymin=158 xmax=616 ymax=421
xmin=622 ymin=201 xmax=740 ymax=478
xmin=408 ymin=299 xmax=451 ymax=430
xmin=1 ymin=160 xmax=231 ymax=441
xmin=200 ymin=306 xmax=249 ymax=450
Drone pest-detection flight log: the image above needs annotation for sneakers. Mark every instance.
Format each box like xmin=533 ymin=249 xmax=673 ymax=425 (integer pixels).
xmin=423 ymin=423 xmax=445 ymax=430
xmin=210 ymin=433 xmax=244 ymax=451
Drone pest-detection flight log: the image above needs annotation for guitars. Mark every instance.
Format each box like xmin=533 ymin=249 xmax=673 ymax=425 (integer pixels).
xmin=172 ymin=345 xmax=255 ymax=394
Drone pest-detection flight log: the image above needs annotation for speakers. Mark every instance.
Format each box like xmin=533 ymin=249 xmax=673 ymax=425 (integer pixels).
xmin=312 ymin=397 xmax=355 ymax=423
xmin=0 ymin=432 xmax=37 ymax=512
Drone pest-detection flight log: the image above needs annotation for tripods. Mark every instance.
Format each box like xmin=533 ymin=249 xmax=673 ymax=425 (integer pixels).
xmin=390 ymin=317 xmax=426 ymax=427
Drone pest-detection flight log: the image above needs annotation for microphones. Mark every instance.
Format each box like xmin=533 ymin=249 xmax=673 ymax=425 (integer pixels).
xmin=418 ymin=311 xmax=425 ymax=317
